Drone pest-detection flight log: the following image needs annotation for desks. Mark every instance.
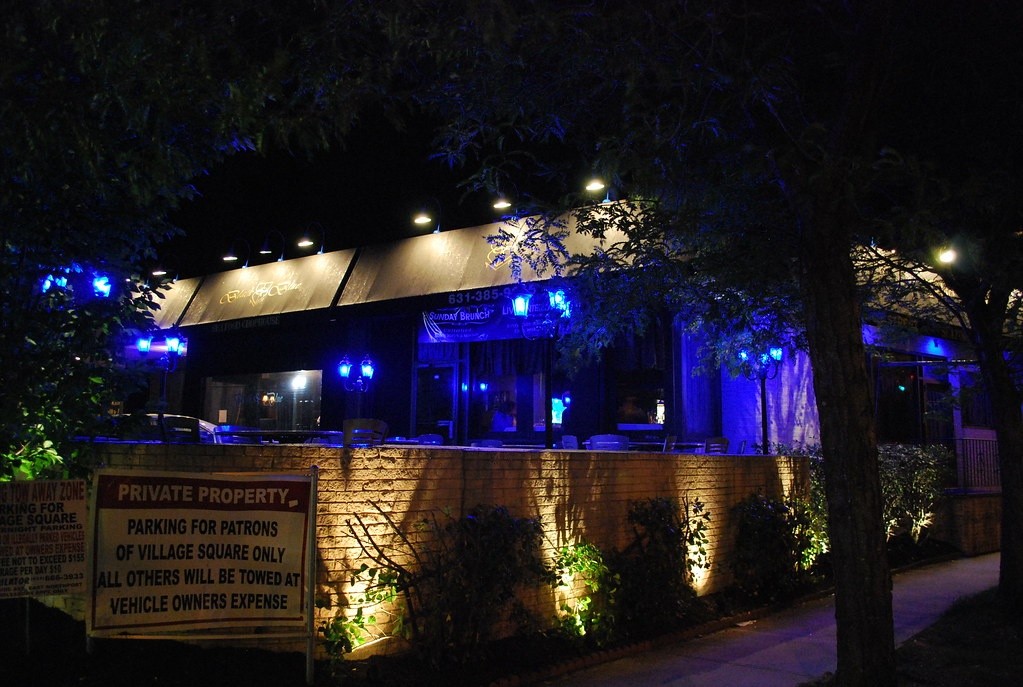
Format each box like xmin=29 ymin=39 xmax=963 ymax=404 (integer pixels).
xmin=233 ymin=429 xmax=343 ymax=446
xmin=582 ymin=441 xmax=705 ymax=453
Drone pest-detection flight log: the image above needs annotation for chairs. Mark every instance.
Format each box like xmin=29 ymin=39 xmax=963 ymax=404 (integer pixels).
xmin=153 ymin=418 xmax=730 ymax=454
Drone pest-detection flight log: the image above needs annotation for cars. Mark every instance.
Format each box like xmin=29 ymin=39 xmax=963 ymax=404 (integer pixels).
xmin=104 ymin=412 xmax=239 ymax=445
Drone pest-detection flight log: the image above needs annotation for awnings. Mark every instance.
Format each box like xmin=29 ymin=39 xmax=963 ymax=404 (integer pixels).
xmin=180 ymin=248 xmax=356 ymax=329
xmin=129 ymin=277 xmax=203 ymax=332
xmin=338 ymin=196 xmax=715 ymax=307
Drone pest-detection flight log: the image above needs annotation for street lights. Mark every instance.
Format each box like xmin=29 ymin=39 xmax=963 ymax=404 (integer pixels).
xmin=138 ymin=324 xmax=186 ymax=435
